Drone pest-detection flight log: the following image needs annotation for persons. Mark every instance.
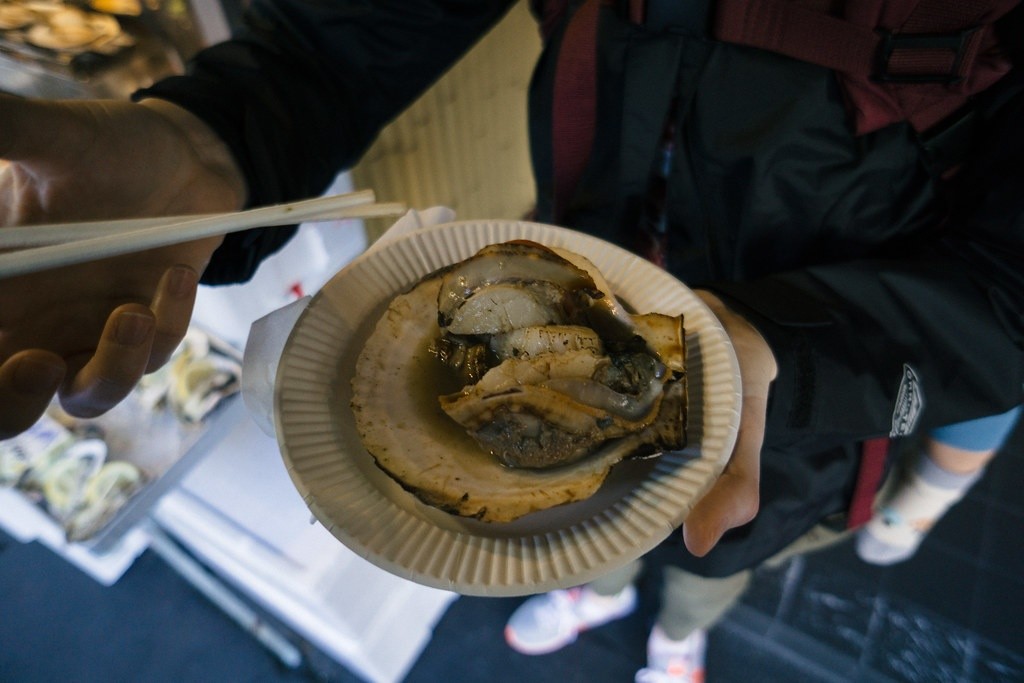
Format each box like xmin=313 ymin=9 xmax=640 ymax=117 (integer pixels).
xmin=0 ymin=0 xmax=1024 ymax=683
xmin=854 ymin=405 xmax=1021 ymax=565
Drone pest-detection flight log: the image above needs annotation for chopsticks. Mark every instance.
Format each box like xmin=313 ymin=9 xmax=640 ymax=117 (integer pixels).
xmin=0 ymin=188 xmax=406 ymax=277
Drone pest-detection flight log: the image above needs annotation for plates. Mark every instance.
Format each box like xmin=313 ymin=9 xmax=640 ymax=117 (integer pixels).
xmin=274 ymin=219 xmax=742 ymax=596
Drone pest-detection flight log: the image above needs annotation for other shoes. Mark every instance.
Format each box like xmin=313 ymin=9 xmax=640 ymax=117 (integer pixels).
xmin=855 ymin=471 xmax=964 ymax=565
xmin=636 ymin=623 xmax=706 ymax=683
xmin=504 ymin=584 xmax=636 ymax=656
